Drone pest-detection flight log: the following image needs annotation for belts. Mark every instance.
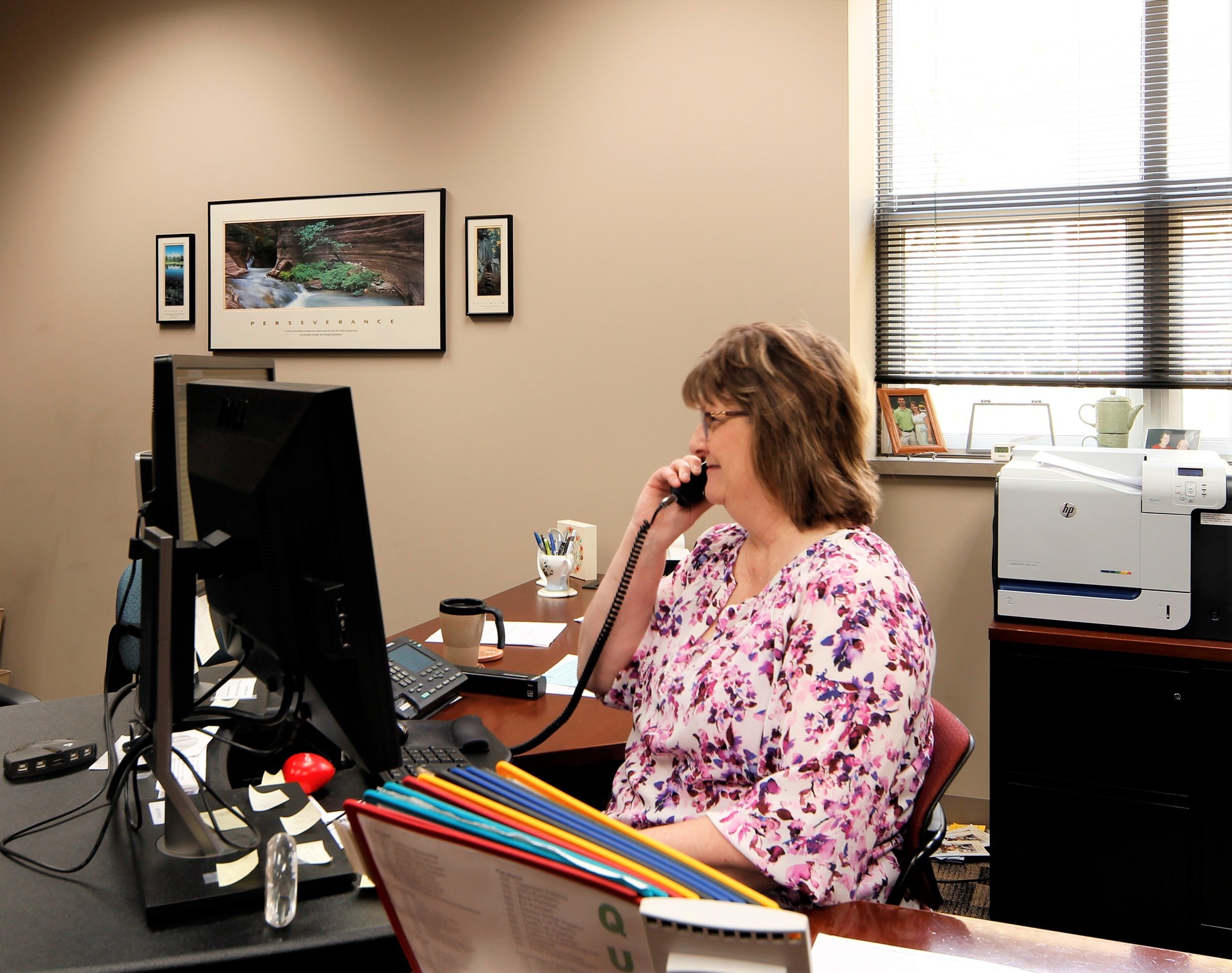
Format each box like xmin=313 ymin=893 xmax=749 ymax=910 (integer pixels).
xmin=900 ymin=429 xmax=913 ymax=433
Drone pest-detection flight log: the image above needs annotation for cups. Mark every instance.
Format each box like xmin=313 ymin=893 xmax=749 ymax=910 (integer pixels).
xmin=540 ymin=553 xmax=574 ymax=591
xmin=439 ymin=597 xmax=505 ymax=669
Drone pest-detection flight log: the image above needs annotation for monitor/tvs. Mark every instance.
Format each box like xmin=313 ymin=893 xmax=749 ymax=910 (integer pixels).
xmin=124 ymin=356 xmax=404 ymax=933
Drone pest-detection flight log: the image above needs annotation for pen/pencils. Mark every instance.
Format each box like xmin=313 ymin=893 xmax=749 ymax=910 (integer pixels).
xmin=534 ymin=532 xmax=544 ymax=553
xmin=557 ymin=529 xmax=576 ymax=556
xmin=549 ymin=533 xmax=556 ymax=555
xmin=543 ymin=539 xmax=551 ymax=556
xmin=540 ymin=534 xmax=551 ymax=555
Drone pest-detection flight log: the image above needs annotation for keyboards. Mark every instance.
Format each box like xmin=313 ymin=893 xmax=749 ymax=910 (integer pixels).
xmin=381 ymin=744 xmax=475 ymax=784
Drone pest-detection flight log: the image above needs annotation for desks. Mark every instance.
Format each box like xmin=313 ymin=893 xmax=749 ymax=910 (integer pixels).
xmin=810 ymin=903 xmax=1232 ymax=973
xmin=387 ymin=572 xmax=634 ymax=812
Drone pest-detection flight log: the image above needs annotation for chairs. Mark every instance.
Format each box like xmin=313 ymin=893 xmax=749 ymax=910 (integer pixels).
xmin=884 ymin=695 xmax=976 ymax=911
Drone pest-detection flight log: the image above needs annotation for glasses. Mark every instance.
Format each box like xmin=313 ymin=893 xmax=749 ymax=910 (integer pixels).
xmin=701 ymin=410 xmax=753 ymax=434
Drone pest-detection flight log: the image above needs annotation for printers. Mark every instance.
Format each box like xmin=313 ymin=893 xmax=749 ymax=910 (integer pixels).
xmin=992 ymin=444 xmax=1232 ymax=644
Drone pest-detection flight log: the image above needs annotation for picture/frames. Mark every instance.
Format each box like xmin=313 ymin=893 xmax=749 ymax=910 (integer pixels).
xmin=465 ymin=214 xmax=513 ymax=316
xmin=155 ymin=233 xmax=196 ymax=326
xmin=877 ymin=387 xmax=949 ymax=456
xmin=208 ymin=188 xmax=445 ymax=354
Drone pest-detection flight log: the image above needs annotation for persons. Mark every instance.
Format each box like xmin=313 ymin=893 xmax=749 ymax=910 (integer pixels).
xmin=893 ymin=395 xmax=939 ymax=449
xmin=575 ymin=324 xmax=935 ymax=904
xmin=1150 ymin=431 xmax=1191 ymax=453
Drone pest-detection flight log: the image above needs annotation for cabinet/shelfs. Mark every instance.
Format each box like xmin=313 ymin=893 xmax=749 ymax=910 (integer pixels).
xmin=990 ymin=620 xmax=1232 ymax=961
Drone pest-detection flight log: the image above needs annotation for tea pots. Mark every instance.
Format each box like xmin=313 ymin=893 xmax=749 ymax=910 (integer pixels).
xmin=1078 ymin=389 xmax=1146 ymax=434
xmin=1081 ymin=434 xmax=1129 ymax=449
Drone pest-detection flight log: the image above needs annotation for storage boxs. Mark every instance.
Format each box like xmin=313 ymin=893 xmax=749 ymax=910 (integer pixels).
xmin=557 ymin=520 xmax=597 ymax=581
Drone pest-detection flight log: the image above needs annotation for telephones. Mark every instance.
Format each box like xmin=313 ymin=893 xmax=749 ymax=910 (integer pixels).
xmin=670 ymin=458 xmax=711 ymax=509
